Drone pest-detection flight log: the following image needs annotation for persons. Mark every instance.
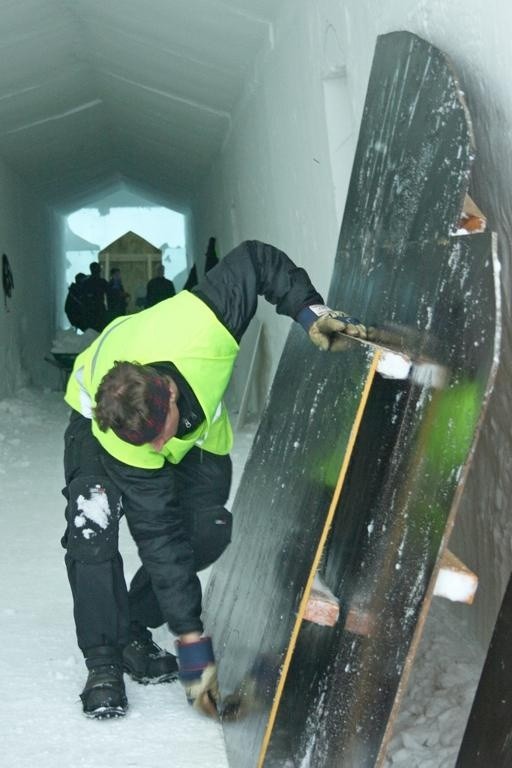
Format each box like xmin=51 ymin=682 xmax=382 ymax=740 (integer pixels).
xmin=65 ymin=262 xmax=175 ymax=335
xmin=61 ymin=241 xmax=367 ymax=718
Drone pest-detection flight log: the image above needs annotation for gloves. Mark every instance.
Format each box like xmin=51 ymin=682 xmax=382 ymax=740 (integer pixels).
xmin=296 ymin=304 xmax=367 ymax=352
xmin=174 ymin=637 xmax=221 ymax=722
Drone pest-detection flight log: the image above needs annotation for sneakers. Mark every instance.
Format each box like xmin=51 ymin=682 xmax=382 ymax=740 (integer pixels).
xmin=79 ymin=636 xmax=179 ymax=720
